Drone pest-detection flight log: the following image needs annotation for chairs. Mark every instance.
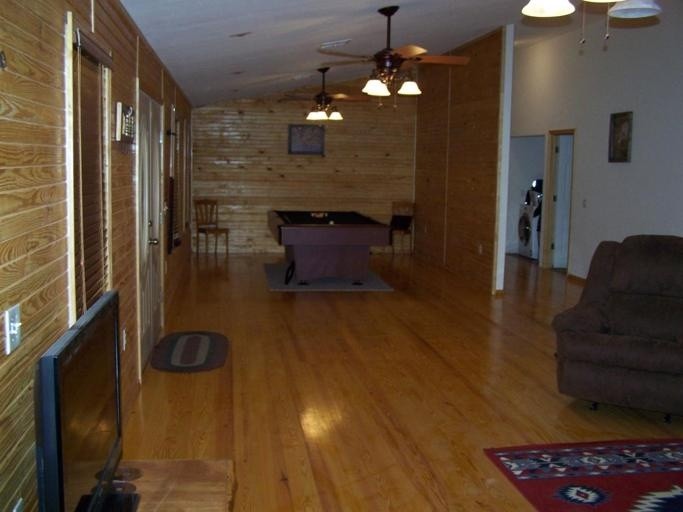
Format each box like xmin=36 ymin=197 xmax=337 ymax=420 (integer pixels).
xmin=193 ymin=199 xmax=229 ymax=263
xmin=387 ymin=199 xmax=417 ymax=258
xmin=550 ymin=235 xmax=682 ymax=424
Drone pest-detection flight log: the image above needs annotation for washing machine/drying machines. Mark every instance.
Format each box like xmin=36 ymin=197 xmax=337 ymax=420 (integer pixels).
xmin=517 ymin=204 xmax=539 ymax=259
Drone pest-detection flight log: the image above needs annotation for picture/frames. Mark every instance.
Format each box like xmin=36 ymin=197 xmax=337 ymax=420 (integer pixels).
xmin=605 ymin=111 xmax=631 ymax=163
xmin=287 ymin=123 xmax=325 ymax=156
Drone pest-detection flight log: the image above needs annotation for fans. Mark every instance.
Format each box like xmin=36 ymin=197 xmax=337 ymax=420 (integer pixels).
xmin=320 ymin=4 xmax=472 ymax=71
xmin=279 ymin=65 xmax=371 ymax=104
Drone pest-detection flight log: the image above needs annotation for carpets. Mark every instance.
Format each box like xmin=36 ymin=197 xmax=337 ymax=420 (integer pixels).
xmin=481 ymin=437 xmax=682 ymax=512
xmin=148 ymin=328 xmax=228 ymax=375
xmin=260 ymin=262 xmax=395 ymax=294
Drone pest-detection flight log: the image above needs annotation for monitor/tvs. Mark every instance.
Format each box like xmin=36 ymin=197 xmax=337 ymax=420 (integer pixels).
xmin=39 ymin=290 xmax=140 ymax=512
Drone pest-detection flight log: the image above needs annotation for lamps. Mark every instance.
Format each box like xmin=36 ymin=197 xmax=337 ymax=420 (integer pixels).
xmin=361 ymin=65 xmax=423 ymax=96
xmin=519 ymin=0 xmax=664 ymax=52
xmin=305 ymin=102 xmax=342 ymax=122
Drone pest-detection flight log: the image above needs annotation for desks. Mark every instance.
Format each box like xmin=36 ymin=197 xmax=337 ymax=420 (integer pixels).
xmin=116 ymin=456 xmax=238 ymax=512
xmin=262 ymin=205 xmax=390 ymax=288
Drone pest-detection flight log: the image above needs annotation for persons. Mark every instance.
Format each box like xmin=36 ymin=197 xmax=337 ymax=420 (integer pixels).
xmin=611 ymin=118 xmax=628 ymax=160
